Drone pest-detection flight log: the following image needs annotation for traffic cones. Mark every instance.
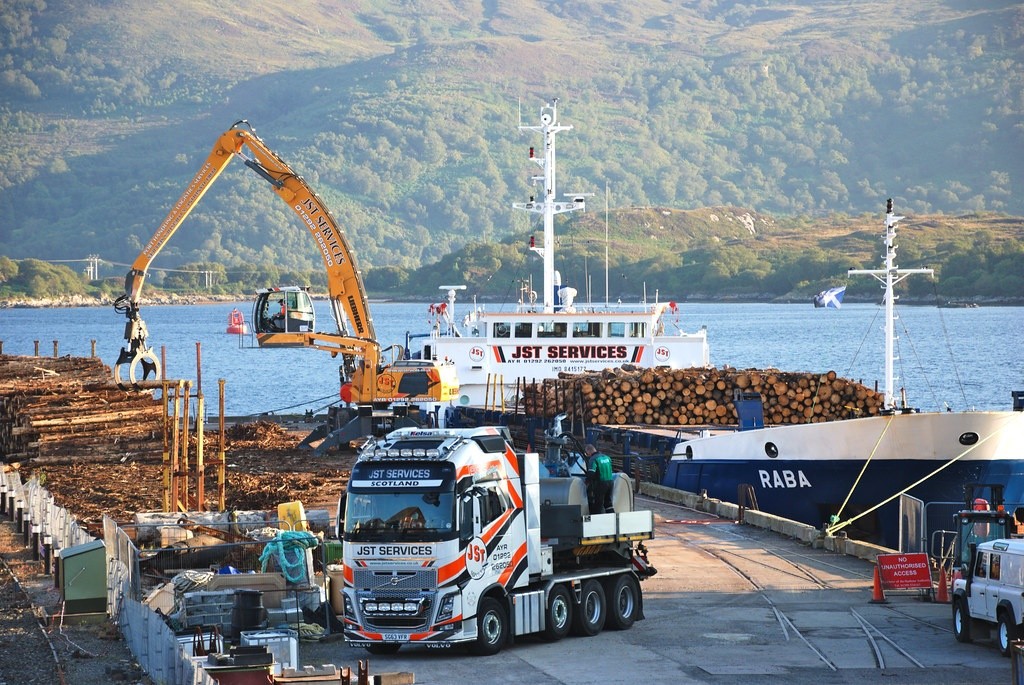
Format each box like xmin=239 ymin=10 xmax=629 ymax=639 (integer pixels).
xmin=872 ymin=566 xmax=885 ymax=601
xmin=936 ymin=566 xmax=950 ymax=601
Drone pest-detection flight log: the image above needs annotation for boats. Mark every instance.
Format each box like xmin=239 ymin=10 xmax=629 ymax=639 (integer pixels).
xmin=395 ymin=97 xmax=710 ymax=429
xmin=662 ymin=197 xmax=1024 ymax=567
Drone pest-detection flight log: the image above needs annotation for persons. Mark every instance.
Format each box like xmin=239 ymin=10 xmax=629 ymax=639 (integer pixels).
xmin=269 ymin=299 xmax=290 ymax=330
xmin=585 ymin=444 xmax=615 ymax=515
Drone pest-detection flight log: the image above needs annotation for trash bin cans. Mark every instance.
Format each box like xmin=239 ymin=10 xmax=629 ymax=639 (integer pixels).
xmin=326 ymin=563 xmax=345 ymax=615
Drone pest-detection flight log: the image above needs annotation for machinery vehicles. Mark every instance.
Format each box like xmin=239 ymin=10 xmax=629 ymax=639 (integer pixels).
xmin=951 ymin=483 xmax=1015 ymax=590
xmin=335 ymin=413 xmax=658 ymax=656
xmin=114 ymin=120 xmax=460 ymax=456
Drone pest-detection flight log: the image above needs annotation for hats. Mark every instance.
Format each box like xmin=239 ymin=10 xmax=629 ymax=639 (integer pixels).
xmin=278 ymin=299 xmax=283 ymax=303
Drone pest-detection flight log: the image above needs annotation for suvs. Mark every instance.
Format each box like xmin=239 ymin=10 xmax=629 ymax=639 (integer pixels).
xmin=952 ymin=539 xmax=1024 ymax=655
